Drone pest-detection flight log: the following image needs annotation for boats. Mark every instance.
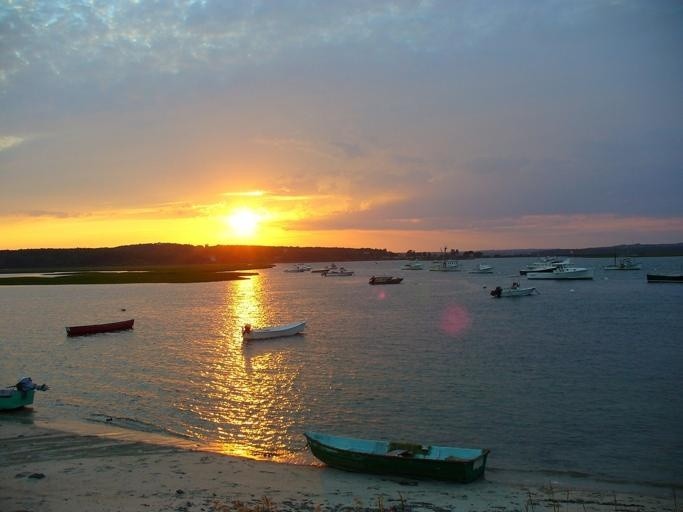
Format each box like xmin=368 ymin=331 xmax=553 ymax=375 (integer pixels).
xmin=604 ymin=253 xmax=642 ymax=270
xmin=241 ymin=320 xmax=306 ymax=340
xmin=520 ymin=256 xmax=593 ymax=280
xmin=469 ymin=261 xmax=494 ymax=273
xmin=429 ymin=260 xmax=459 ymax=271
xmin=284 ymin=262 xmax=354 ymax=276
xmin=646 ymin=274 xmax=683 ymax=283
xmin=490 ymin=280 xmax=537 ymax=297
xmin=64 ymin=318 xmax=134 ymax=337
xmin=401 ymin=258 xmax=426 ymax=271
xmin=1 ymin=388 xmax=35 ymax=410
xmin=368 ymin=275 xmax=404 ymax=284
xmin=302 ymin=429 xmax=491 ymax=485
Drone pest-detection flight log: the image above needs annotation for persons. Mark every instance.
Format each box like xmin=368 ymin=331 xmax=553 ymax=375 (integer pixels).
xmin=509 ymin=281 xmax=517 ymax=290
